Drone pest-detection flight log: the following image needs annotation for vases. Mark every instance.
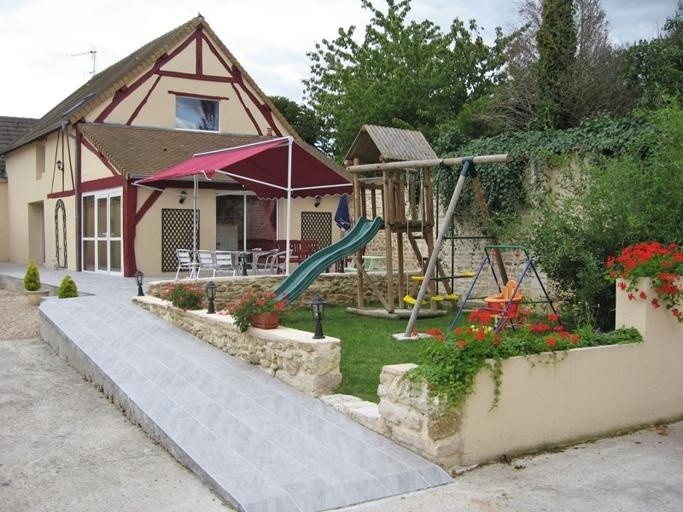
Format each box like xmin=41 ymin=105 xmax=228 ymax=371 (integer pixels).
xmin=247 ymin=310 xmax=281 ymax=329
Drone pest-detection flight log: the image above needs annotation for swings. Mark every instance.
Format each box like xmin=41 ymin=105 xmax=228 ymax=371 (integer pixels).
xmin=485 ymin=247 xmax=524 ymax=317
xmin=404 ymin=166 xmax=430 ymax=306
xmin=432 ymin=167 xmax=462 ymax=301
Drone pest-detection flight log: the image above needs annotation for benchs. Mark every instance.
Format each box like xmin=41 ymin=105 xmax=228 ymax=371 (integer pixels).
xmin=347 ymin=255 xmax=387 ymax=271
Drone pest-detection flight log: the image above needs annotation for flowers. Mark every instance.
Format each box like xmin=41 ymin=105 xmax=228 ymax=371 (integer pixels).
xmin=226 ymin=286 xmax=288 ymax=333
xmin=163 ymin=281 xmax=206 ymax=312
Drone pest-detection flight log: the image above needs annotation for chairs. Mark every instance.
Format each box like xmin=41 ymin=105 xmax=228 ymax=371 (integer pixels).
xmin=172 ymin=239 xmax=328 ymax=279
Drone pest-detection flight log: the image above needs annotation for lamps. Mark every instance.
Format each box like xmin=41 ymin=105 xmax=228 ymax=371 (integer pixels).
xmin=308 ymin=294 xmax=329 ymax=338
xmin=133 ymin=270 xmax=145 ymax=296
xmin=205 ymin=280 xmax=218 ymax=314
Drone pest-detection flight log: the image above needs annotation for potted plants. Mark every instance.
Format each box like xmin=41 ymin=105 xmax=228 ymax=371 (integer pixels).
xmin=22 ymin=257 xmax=50 ymax=306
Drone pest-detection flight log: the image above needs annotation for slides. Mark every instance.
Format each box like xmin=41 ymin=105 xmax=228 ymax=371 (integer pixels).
xmin=269 ymin=216 xmax=384 ymax=307
